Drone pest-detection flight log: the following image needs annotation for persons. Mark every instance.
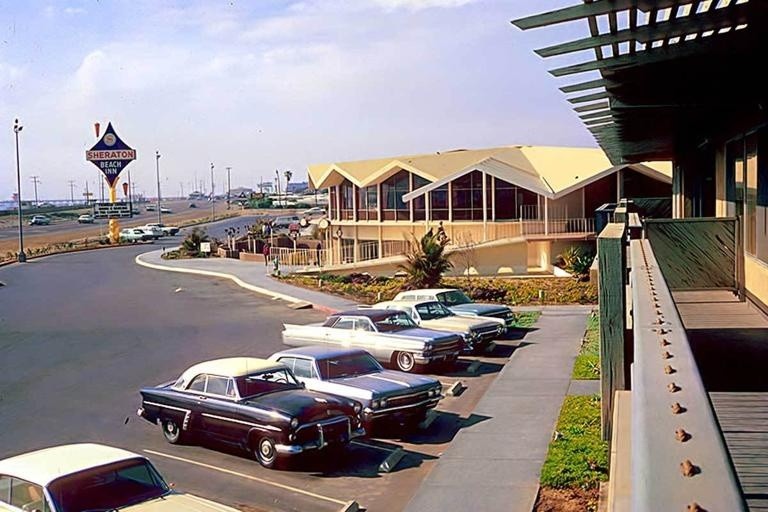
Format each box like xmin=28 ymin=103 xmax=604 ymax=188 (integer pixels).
xmin=273 ymin=256 xmax=280 ymax=271
xmin=263 ymin=242 xmax=270 ymax=266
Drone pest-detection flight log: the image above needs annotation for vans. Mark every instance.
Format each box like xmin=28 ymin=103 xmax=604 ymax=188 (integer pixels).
xmin=272 ymin=215 xmax=299 ymax=229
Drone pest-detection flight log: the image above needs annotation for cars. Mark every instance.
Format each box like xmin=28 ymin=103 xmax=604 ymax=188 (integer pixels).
xmin=76 ymin=214 xmax=95 ymax=225
xmin=365 ymin=300 xmax=507 ymax=362
xmin=99 ymin=221 xmax=179 ymax=244
xmin=160 ymin=205 xmax=171 ymax=212
xmin=145 ymin=204 xmax=155 ymax=210
xmin=396 ymin=286 xmax=514 ymax=332
xmin=132 ymin=208 xmax=141 ymax=215
xmin=305 ymin=207 xmax=325 ymax=216
xmin=189 ymin=203 xmax=196 ymax=208
xmin=279 ymin=307 xmax=463 ymax=376
xmin=136 ymin=356 xmax=368 ymax=471
xmin=0 ymin=443 xmax=242 ymax=512
xmin=265 ymin=345 xmax=442 ymax=441
xmin=32 ymin=215 xmax=49 ymax=225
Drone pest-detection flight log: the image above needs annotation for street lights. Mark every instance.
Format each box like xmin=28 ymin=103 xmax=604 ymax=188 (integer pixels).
xmin=154 ymin=148 xmax=162 ymax=224
xmin=275 ymin=169 xmax=281 ymax=207
xmin=211 ymin=162 xmax=215 ymax=221
xmin=225 ymin=167 xmax=232 ymax=210
xmin=13 ymin=118 xmax=26 ymax=263
